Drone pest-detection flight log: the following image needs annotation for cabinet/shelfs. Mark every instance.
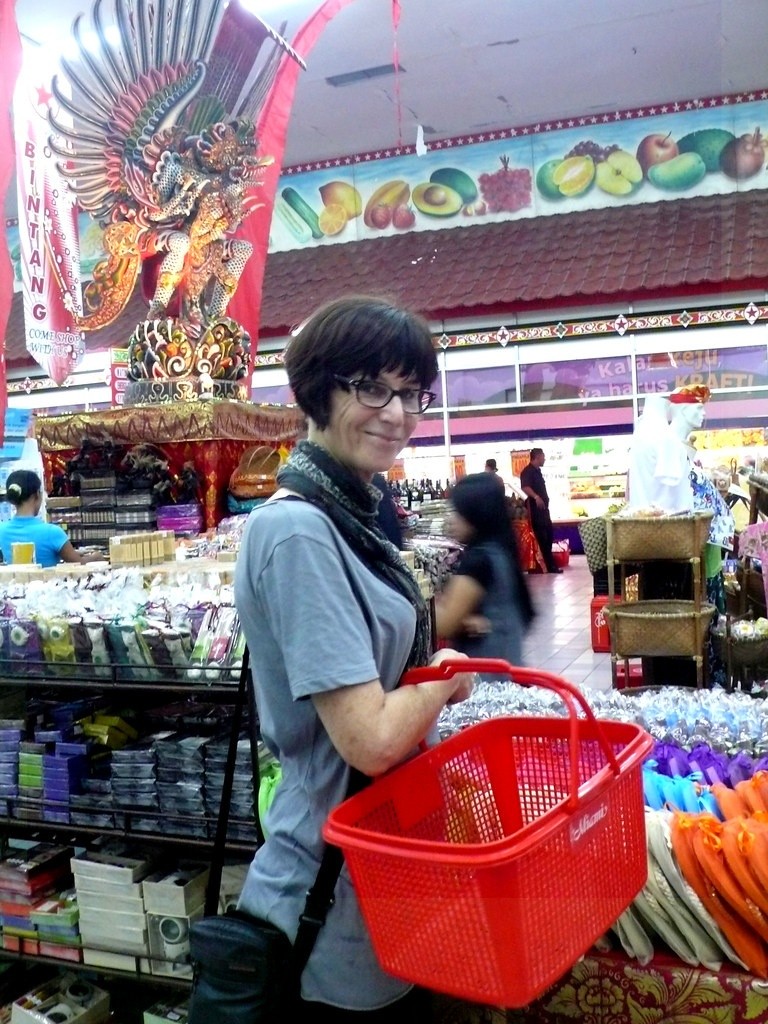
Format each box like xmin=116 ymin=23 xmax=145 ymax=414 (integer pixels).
xmin=0 ymin=594 xmax=437 ymax=1024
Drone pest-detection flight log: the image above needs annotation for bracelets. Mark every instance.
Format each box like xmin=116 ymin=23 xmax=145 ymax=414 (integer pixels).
xmin=534 ymin=494 xmax=540 ymax=499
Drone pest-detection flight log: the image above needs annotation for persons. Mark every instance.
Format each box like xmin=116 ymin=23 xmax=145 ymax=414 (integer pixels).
xmin=227 ymin=292 xmax=476 ymax=1022
xmin=431 ymin=470 xmax=525 ymax=675
xmin=665 ymin=384 xmax=709 ymax=450
xmin=0 ymin=470 xmax=104 ymax=565
xmin=485 ymin=460 xmax=497 ymax=474
xmin=519 ymin=448 xmax=563 ymax=574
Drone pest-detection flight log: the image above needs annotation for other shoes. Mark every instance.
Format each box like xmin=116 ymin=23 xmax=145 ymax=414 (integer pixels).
xmin=549 ymin=568 xmax=563 ymax=572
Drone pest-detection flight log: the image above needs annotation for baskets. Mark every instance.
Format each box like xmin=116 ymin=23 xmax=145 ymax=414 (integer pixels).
xmin=319 ymin=661 xmax=656 ymax=1006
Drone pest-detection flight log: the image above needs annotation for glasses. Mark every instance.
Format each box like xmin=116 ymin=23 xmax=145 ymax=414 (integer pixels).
xmin=333 ymin=373 xmax=437 ymax=414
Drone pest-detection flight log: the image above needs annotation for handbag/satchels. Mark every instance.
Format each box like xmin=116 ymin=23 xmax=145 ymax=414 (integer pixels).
xmin=185 ymin=910 xmax=302 ymax=1024
